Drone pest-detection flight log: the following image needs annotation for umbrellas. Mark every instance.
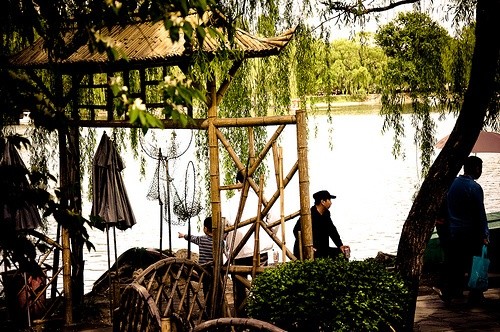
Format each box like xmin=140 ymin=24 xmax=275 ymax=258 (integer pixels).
xmin=434 ymin=130 xmax=500 ymax=156
xmin=90 ymin=131 xmax=136 ymax=316
xmin=0 ymin=133 xmax=44 ymax=276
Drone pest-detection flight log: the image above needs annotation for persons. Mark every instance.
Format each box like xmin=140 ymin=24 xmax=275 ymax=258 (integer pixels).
xmin=291 ymin=190 xmax=349 ymax=263
xmin=221 ymin=214 xmax=272 ymax=320
xmin=433 ymin=157 xmax=491 ymax=310
xmin=176 ymin=217 xmax=224 ymax=320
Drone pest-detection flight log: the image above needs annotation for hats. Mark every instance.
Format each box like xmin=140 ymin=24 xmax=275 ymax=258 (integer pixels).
xmin=313 ymin=190 xmax=336 ymax=201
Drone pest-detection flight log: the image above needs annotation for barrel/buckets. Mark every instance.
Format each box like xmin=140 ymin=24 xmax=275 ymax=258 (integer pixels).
xmin=0 ymin=269 xmax=47 ymax=326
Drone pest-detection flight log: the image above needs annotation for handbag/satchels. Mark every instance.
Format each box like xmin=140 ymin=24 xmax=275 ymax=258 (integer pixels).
xmin=467 ymin=245 xmax=490 ymax=291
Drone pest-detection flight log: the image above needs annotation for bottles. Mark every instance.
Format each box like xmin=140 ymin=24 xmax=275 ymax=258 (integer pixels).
xmin=344 ymin=246 xmax=350 ymax=258
